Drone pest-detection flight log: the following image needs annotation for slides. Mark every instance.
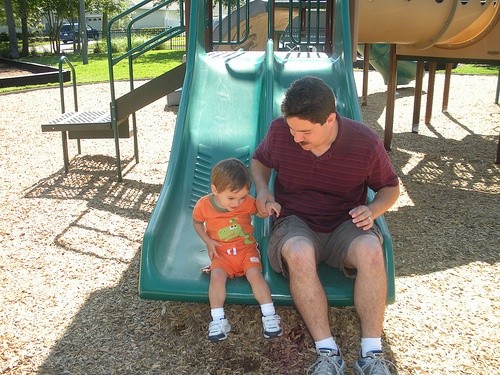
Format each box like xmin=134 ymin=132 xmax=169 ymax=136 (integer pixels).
xmin=358 ymin=43 xmax=425 ymax=86
xmin=137 ymin=0 xmax=395 ymax=305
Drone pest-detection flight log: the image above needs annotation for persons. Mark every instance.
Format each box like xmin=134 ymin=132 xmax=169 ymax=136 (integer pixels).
xmin=192 ymin=158 xmax=283 ymax=342
xmin=251 ymin=76 xmax=400 ymax=375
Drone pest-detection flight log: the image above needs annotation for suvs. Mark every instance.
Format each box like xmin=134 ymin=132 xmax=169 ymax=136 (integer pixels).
xmin=59 ymin=23 xmax=100 ymax=44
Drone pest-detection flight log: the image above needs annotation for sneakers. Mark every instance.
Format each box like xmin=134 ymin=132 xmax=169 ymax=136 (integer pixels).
xmin=208 ymin=316 xmax=231 ymax=343
xmin=355 ymin=348 xmax=398 ymax=375
xmin=262 ymin=313 xmax=282 ymax=339
xmin=307 ymin=344 xmax=345 ymax=375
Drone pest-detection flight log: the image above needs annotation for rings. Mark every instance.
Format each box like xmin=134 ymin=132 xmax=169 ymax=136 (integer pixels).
xmin=368 ymin=216 xmax=372 ymax=222
